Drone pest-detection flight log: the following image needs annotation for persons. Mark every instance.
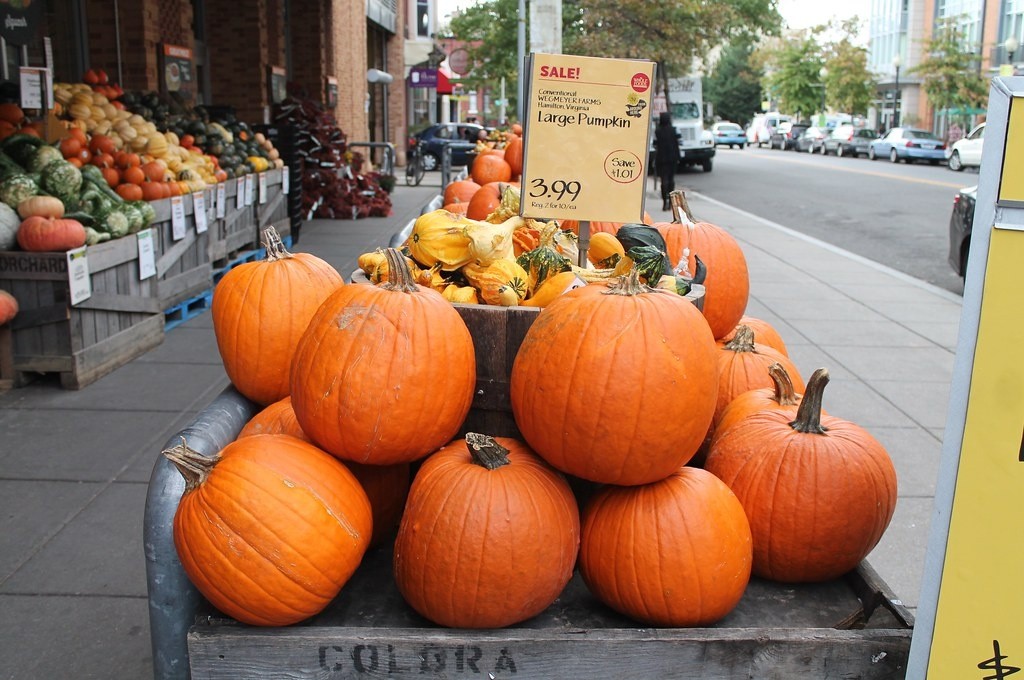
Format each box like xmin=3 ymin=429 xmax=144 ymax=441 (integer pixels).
xmin=457 ymin=118 xmax=482 ymax=142
xmin=652 ymin=112 xmax=683 ymax=211
xmin=948 ymin=122 xmax=962 ymax=147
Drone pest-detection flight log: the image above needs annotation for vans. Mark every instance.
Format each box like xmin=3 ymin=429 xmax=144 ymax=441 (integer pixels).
xmin=746 ymin=114 xmax=791 ymax=148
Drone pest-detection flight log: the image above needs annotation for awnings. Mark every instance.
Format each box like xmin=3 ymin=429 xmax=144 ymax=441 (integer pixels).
xmin=435 ymin=69 xmax=458 ymax=94
xmin=938 ymin=104 xmax=988 ymax=116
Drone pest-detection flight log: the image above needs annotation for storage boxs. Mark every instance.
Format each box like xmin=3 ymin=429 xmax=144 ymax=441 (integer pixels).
xmin=256 ymin=246 xmax=267 ymax=260
xmin=227 ymin=257 xmax=245 ymax=269
xmin=281 ymin=235 xmax=293 ymax=248
xmin=164 ymin=302 xmax=185 ymax=331
xmin=240 ymin=249 xmax=259 ymax=261
xmin=184 ymin=288 xmax=213 ymax=321
xmin=211 ymin=266 xmax=230 ymax=286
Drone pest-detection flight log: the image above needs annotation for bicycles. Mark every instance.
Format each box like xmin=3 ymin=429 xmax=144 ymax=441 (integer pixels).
xmin=405 ymin=134 xmax=426 ymax=187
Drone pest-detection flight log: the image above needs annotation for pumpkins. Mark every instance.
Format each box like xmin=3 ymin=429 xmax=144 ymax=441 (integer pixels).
xmin=0 ymin=288 xmax=19 ymax=326
xmin=0 ymin=68 xmax=283 ymax=251
xmin=162 ymin=121 xmax=899 ymax=630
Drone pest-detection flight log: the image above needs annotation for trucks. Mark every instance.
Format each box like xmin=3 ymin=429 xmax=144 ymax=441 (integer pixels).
xmin=647 ymin=77 xmax=716 ymax=176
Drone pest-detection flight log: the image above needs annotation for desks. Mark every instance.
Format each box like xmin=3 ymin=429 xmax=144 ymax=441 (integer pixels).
xmin=257 ymin=168 xmax=292 ymax=247
xmin=185 ymin=485 xmax=915 ymax=680
xmin=0 ymin=227 xmax=165 ymax=391
xmin=149 ymin=189 xmax=214 ymax=311
xmin=212 ymin=174 xmax=257 ymax=263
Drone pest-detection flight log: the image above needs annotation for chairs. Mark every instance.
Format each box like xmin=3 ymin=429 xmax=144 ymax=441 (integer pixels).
xmin=464 ymin=130 xmax=471 ymax=140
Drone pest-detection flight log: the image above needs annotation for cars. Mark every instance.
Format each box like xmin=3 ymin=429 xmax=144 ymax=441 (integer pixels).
xmin=947 ymin=122 xmax=987 ymax=172
xmin=711 ymin=123 xmax=748 ymax=150
xmin=820 ymin=124 xmax=880 ymax=158
xmin=947 ymin=185 xmax=978 ymax=285
xmin=795 ymin=125 xmax=833 ymax=154
xmin=867 ymin=128 xmax=948 ymax=166
xmin=406 ymin=124 xmax=485 ymax=172
xmin=768 ymin=122 xmax=811 ymax=150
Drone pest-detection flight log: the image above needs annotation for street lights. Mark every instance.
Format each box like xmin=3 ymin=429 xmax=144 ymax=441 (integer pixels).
xmin=1005 ymin=35 xmax=1019 ymax=65
xmin=892 ymin=53 xmax=904 ymax=128
